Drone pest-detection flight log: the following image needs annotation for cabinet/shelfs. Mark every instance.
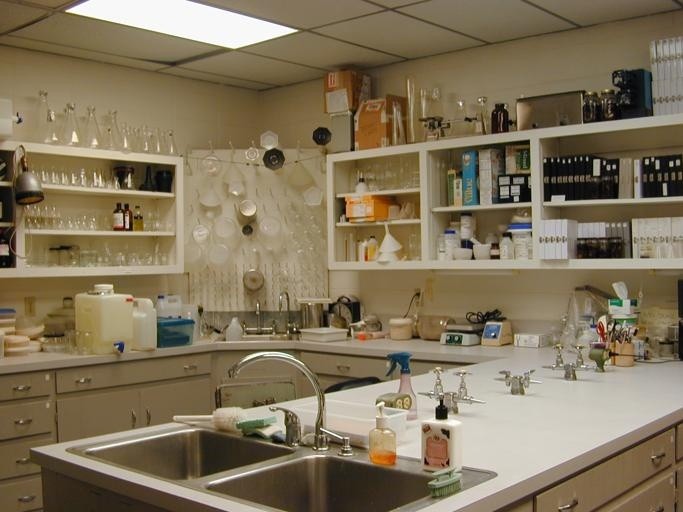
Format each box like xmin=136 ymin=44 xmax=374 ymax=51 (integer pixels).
xmin=55 ymin=347 xmax=214 ymax=444
xmin=214 ymin=348 xmax=298 ymax=409
xmin=424 ymin=129 xmax=533 ymax=270
xmin=325 ymin=143 xmax=428 ymax=271
xmin=674 ymin=419 xmax=683 ymax=512
xmin=533 ymin=114 xmax=683 ymax=270
xmin=0 ymin=140 xmax=185 ymax=279
xmin=498 ymin=497 xmax=534 ymax=512
xmin=529 ymin=423 xmax=682 ymax=512
xmin=298 ymin=352 xmax=467 ymax=398
xmin=2 ymin=371 xmax=55 ymax=511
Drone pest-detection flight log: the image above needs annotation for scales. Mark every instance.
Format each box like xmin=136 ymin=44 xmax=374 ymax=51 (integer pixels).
xmin=438 ymin=323 xmax=484 ymax=347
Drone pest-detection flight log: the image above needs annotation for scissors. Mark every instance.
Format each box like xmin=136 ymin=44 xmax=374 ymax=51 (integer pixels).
xmin=607 ymin=319 xmax=623 ymax=336
xmin=596 ymin=321 xmax=606 ymax=342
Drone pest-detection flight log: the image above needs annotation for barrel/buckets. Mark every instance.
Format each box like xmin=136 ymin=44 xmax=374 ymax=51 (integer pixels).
xmin=153 ymin=294 xmax=183 ymax=317
xmin=132 ymin=297 xmax=158 ymax=352
xmin=73 ymin=282 xmax=135 ymax=355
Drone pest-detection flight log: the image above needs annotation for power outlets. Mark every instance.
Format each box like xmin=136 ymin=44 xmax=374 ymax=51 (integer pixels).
xmin=413 ymin=288 xmax=423 ymax=307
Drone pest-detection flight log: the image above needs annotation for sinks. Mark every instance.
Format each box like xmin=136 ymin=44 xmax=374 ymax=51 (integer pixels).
xmin=64 ymin=424 xmax=297 ymax=488
xmin=243 ymin=327 xmax=275 ymax=337
xmin=196 ymin=443 xmax=499 ymax=512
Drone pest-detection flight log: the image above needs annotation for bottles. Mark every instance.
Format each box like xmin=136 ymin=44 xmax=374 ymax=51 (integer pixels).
xmin=105 ymin=109 xmax=123 ymax=151
xmin=83 ymin=106 xmax=103 ymax=147
xmin=34 ymin=89 xmax=58 ymax=144
xmin=132 ymin=205 xmax=143 ymax=230
xmin=120 ymin=120 xmax=177 ymax=155
xmin=367 ymin=235 xmax=379 ymax=261
xmin=360 ymin=238 xmax=368 ymax=260
xmin=387 ymin=72 xmax=509 ymax=147
xmin=438 ymin=211 xmax=515 ymax=262
xmin=29 ymin=165 xmax=120 ymax=189
xmin=123 ymin=204 xmax=133 ymax=231
xmin=43 ymin=242 xmax=167 ymax=267
xmin=582 ymin=87 xmax=619 ymax=123
xmin=25 ymin=205 xmax=96 ymax=231
xmin=61 ymin=102 xmax=82 ymax=146
xmin=112 ymin=202 xmax=124 ymax=230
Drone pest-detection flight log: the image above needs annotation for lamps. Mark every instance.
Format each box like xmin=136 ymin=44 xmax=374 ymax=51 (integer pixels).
xmin=12 ymin=144 xmax=45 ymax=205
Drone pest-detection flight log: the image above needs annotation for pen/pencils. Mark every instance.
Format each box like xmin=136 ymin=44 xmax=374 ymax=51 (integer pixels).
xmin=616 ymin=327 xmax=639 ymax=343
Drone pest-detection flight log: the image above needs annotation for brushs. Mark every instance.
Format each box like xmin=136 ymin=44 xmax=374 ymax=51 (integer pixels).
xmin=171 ymin=406 xmax=248 ymax=432
xmin=427 ymin=465 xmax=462 ymax=497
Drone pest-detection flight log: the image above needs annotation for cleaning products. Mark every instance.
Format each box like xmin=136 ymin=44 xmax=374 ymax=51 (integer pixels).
xmin=384 ymin=350 xmax=418 ymax=422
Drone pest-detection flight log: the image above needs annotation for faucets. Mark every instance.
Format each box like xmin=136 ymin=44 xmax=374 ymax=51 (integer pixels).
xmin=278 ymin=290 xmax=291 ymax=334
xmin=255 ymin=299 xmax=263 ymax=332
xmin=228 ymin=351 xmax=330 ymax=452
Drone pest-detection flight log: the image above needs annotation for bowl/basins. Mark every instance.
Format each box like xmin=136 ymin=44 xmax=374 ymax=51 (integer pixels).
xmin=185 ymin=178 xmax=285 ymax=292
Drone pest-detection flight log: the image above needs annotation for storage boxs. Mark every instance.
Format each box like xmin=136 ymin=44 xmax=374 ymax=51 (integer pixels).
xmin=328 ymin=113 xmax=356 ymax=156
xmin=157 ymin=316 xmax=197 ymax=349
xmin=323 ymin=69 xmax=372 ymax=113
xmin=353 ymin=94 xmax=408 ymax=151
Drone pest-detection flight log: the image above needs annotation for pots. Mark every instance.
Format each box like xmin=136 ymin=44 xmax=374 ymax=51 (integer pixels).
xmin=47 ymin=296 xmax=75 ymax=318
